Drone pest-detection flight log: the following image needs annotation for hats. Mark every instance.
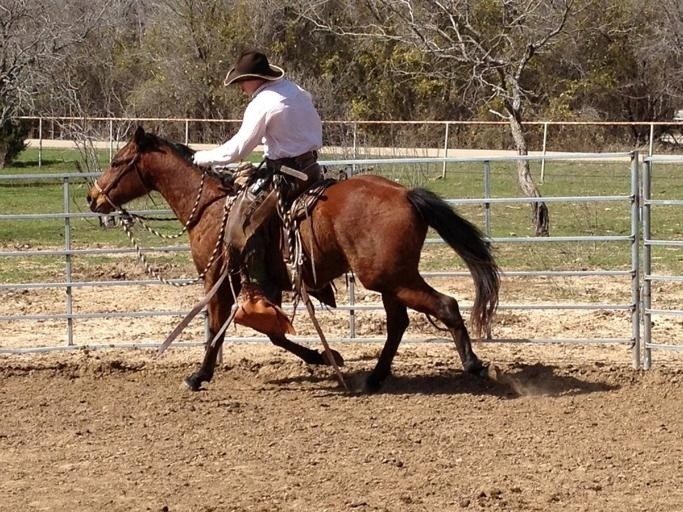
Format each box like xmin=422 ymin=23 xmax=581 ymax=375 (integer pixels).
xmin=223 ymin=51 xmax=285 ymax=87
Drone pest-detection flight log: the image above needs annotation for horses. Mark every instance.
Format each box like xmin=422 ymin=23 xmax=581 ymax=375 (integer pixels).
xmin=86 ymin=124 xmax=505 ymax=397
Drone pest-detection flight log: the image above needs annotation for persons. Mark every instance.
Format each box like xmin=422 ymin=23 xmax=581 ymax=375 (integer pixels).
xmin=174 ymin=49 xmax=322 ymax=309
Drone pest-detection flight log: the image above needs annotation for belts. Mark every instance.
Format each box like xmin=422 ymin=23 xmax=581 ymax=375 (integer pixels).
xmin=265 ymin=151 xmax=315 ymax=182
